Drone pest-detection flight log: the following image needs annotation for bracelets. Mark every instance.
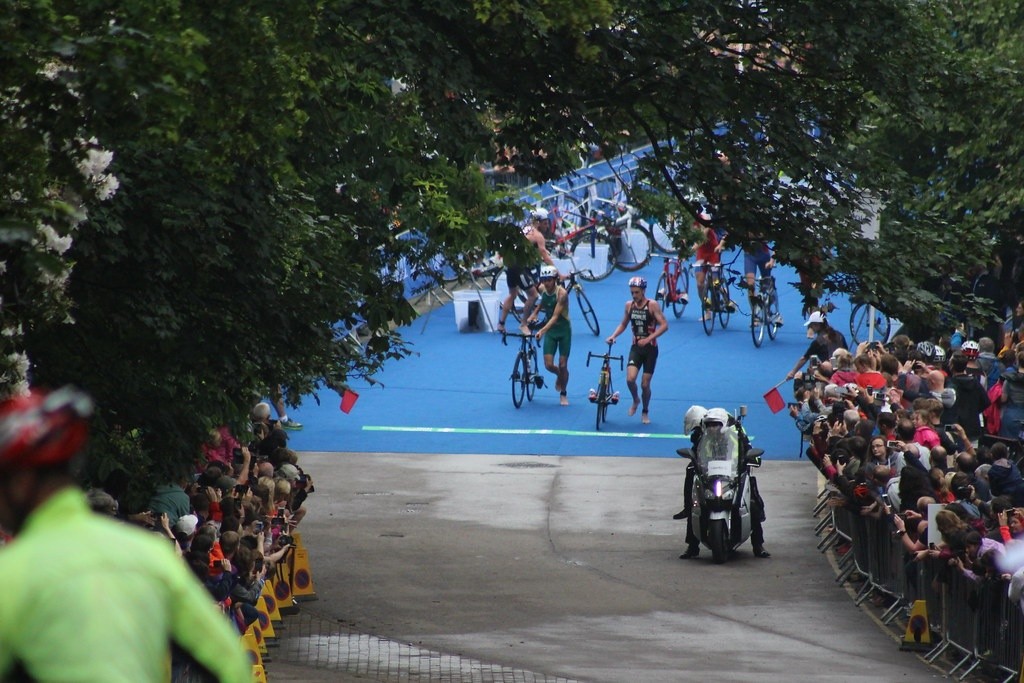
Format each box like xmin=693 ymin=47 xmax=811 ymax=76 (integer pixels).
xmin=966 ymin=445 xmax=973 ymax=451
xmin=897 ymin=530 xmax=908 ymax=536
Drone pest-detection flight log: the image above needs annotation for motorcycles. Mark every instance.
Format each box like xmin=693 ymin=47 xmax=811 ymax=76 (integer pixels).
xmin=676 ymin=425 xmax=766 ymax=563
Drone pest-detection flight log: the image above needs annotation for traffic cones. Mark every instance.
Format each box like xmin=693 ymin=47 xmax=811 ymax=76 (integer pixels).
xmin=293 ymin=531 xmax=319 ymax=602
xmin=261 ymin=579 xmax=287 ymax=630
xmin=254 ymin=597 xmax=281 ymax=648
xmin=899 ymin=599 xmax=933 ymax=653
xmin=240 ymin=626 xmax=266 ymax=683
xmin=272 ymin=564 xmax=300 ymax=615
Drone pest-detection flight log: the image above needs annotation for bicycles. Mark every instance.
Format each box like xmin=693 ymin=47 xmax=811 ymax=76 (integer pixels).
xmin=586 ymin=339 xmax=624 ymax=431
xmin=490 ymin=174 xmax=681 ymax=315
xmin=849 ymin=300 xmax=892 ymax=347
xmin=499 ymin=328 xmax=548 ymax=410
xmin=508 ymin=269 xmax=602 ymax=337
xmin=691 ymin=261 xmax=730 ymax=337
xmin=650 ymin=253 xmax=690 ymax=319
xmin=738 ymin=275 xmax=779 ymax=348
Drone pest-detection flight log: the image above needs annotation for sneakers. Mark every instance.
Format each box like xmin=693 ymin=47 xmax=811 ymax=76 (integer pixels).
xmin=749 ymin=320 xmax=759 ymax=328
xmin=658 ymin=288 xmax=666 ymax=299
xmin=680 ymin=293 xmax=689 ymax=306
xmin=279 ymin=417 xmax=304 ymax=431
xmin=774 ymin=316 xmax=783 ymax=328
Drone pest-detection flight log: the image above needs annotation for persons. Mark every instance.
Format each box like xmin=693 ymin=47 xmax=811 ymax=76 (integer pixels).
xmin=0 ymin=379 xmax=311 ymax=683
xmin=384 ymin=117 xmax=1023 ymax=683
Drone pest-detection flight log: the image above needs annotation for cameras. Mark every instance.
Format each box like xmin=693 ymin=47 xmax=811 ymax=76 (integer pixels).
xmin=279 ymin=535 xmax=293 ymax=547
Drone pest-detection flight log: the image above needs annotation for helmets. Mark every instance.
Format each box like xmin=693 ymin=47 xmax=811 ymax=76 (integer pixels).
xmin=540 ymin=265 xmax=558 ymax=279
xmin=933 ymin=344 xmax=946 ymax=363
xmin=917 ymin=341 xmax=936 ymax=362
xmin=0 ymin=386 xmax=88 ymax=511
xmin=684 ymin=404 xmax=730 ymax=435
xmin=530 ymin=207 xmax=549 ymax=223
xmin=628 ymin=277 xmax=648 ymax=288
xmin=961 ymin=340 xmax=981 ymax=361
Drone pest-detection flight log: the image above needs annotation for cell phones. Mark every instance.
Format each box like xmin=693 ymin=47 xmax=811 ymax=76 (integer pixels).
xmin=834 ymin=387 xmax=849 ymax=393
xmin=235 ymin=485 xmax=248 ymax=493
xmin=272 ymin=518 xmax=285 ymax=525
xmin=945 ymin=424 xmax=956 ymax=431
xmin=255 ymin=558 xmax=262 ymax=574
xmin=930 ymin=542 xmax=935 ymax=550
xmin=278 ymin=508 xmax=284 ymax=517
xmin=867 ymin=386 xmax=872 ymax=397
xmin=887 ymin=440 xmax=899 ymax=448
xmin=868 ymin=342 xmax=879 ymax=349
xmin=152 ymin=513 xmax=163 ymax=520
xmin=1006 ymin=509 xmax=1015 ymax=517
xmin=214 ymin=560 xmax=221 ymax=567
xmin=882 ymin=494 xmax=891 ymax=507
xmin=831 ymin=357 xmax=838 ymax=371
xmin=197 ymin=486 xmax=208 ymax=493
xmin=897 ymin=513 xmax=907 ymax=520
xmin=875 ymin=392 xmax=886 ymax=400
xmin=811 ymin=355 xmax=818 ymax=368
xmin=788 ymin=403 xmax=802 ymax=410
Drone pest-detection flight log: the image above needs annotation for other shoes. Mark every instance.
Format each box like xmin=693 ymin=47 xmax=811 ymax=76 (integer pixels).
xmin=673 ymin=508 xmax=688 ymax=519
xmin=699 ymin=312 xmax=711 ymax=321
xmin=679 ymin=546 xmax=700 ymax=559
xmin=753 ymin=545 xmax=772 ymax=557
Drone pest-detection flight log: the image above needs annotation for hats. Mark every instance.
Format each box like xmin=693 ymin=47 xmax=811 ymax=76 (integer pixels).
xmin=277 ymin=464 xmax=300 ymax=480
xmin=176 ymin=514 xmax=198 ymax=536
xmin=804 ymin=310 xmax=826 ymax=326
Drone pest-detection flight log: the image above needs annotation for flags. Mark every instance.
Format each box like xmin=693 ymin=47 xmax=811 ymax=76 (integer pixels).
xmin=764 ymin=387 xmax=785 ymax=413
xmin=340 ymin=389 xmax=360 ymax=413
